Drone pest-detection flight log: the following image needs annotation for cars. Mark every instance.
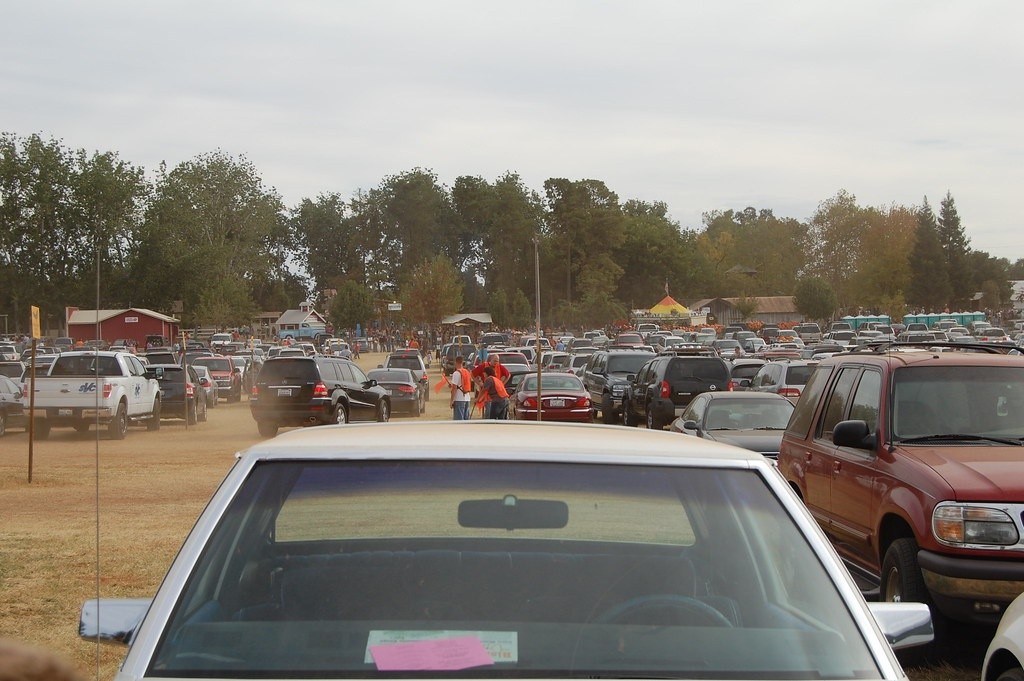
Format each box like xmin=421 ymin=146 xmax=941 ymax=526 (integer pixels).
xmin=980 ymin=590 xmax=1024 ymax=680
xmin=670 ymin=391 xmax=796 ymax=506
xmin=377 ymin=348 xmax=430 ymax=401
xmin=366 ymin=368 xmax=425 ymax=417
xmin=78 ymin=419 xmax=935 ymax=679
xmin=439 ymin=319 xmax=1024 ymax=420
xmin=350 ymin=339 xmax=370 ymax=353
xmin=0 ymin=333 xmax=352 ymax=440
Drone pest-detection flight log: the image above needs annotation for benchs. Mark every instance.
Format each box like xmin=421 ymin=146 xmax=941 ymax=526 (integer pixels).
xmin=237 ymin=550 xmax=718 ymax=622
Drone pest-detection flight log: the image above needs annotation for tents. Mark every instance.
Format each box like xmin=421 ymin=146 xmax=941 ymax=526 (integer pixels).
xmin=650 ymin=296 xmax=690 ymax=315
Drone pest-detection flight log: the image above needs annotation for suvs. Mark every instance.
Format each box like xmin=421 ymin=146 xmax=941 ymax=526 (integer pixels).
xmin=778 ymin=343 xmax=1024 ymax=624
xmin=249 ymin=354 xmax=391 ymax=436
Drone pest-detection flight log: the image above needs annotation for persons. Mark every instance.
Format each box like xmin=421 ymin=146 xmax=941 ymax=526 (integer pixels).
xmin=0 ymin=302 xmax=1024 ymax=420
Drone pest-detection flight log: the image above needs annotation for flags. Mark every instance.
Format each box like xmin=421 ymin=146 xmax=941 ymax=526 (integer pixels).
xmin=435 ymin=374 xmax=447 ymax=393
xmin=664 ymin=281 xmax=668 ymax=293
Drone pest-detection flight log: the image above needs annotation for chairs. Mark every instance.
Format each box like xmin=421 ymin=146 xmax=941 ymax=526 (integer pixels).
xmin=261 ymin=566 xmax=429 ymax=620
xmin=708 ymin=410 xmax=730 ymax=426
xmin=601 ymin=560 xmax=741 ymax=628
xmin=898 ymin=400 xmax=950 ymax=433
